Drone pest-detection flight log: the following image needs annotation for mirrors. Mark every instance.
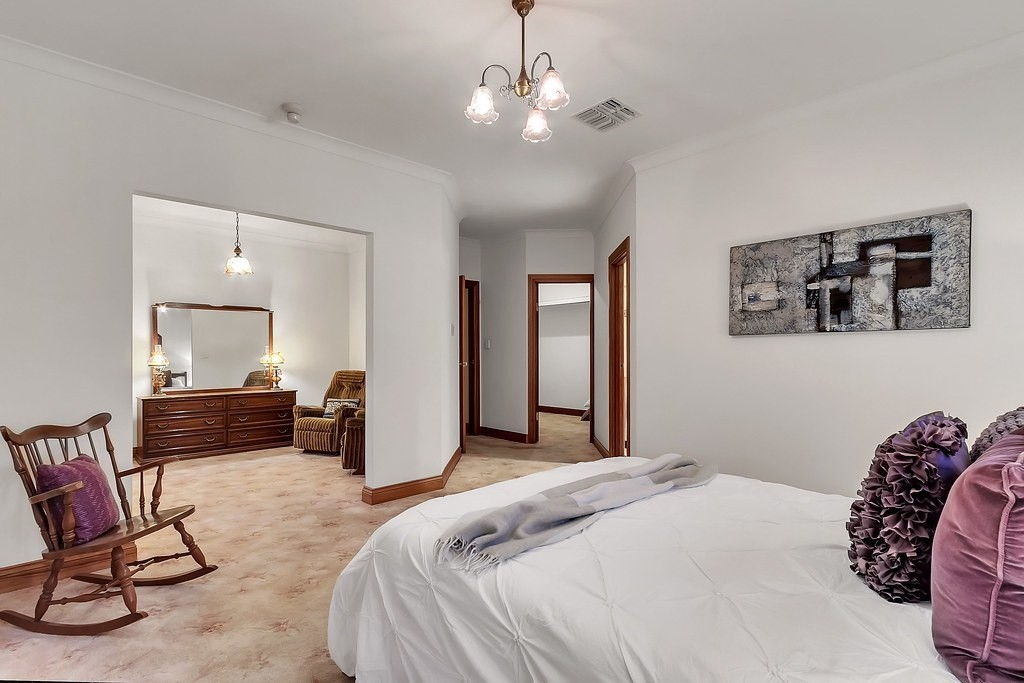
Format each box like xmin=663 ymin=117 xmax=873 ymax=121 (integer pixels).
xmin=151 ymin=301 xmax=274 ymax=395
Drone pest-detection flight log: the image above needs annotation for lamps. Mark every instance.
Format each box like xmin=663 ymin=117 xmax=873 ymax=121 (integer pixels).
xmin=464 ymin=0 xmax=571 ymax=145
xmin=270 ymin=345 xmax=285 ymax=391
xmin=148 ymin=344 xmax=169 ymax=397
xmin=225 ymin=212 xmax=253 ymax=275
xmin=260 ymin=345 xmax=270 ymax=387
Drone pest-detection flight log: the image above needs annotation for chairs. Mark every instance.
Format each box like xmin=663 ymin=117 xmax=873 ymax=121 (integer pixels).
xmin=2 ymin=411 xmax=218 ymax=638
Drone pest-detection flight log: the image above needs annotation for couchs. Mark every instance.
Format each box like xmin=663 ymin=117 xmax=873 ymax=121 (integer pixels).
xmin=340 ymin=409 xmax=365 ymax=476
xmin=291 ymin=369 xmax=366 ymax=456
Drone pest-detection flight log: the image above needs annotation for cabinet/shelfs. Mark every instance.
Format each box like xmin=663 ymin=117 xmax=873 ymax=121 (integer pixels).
xmin=134 ymin=388 xmax=298 ymax=465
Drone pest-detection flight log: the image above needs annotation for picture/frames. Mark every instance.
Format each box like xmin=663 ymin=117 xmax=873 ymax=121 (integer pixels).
xmin=729 ymin=210 xmax=972 ymax=336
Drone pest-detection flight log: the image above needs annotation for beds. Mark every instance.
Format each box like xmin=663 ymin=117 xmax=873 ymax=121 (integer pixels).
xmin=326 ymin=453 xmax=964 ymax=683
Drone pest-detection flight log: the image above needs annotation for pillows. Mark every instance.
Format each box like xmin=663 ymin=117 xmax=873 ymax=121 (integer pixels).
xmin=843 ymin=410 xmax=971 ymax=606
xmin=36 ymin=452 xmax=121 ymax=544
xmin=970 ymin=404 xmax=1024 ymax=464
xmin=322 ymin=398 xmax=361 ymax=419
xmin=927 ymin=426 xmax=1024 ymax=683
